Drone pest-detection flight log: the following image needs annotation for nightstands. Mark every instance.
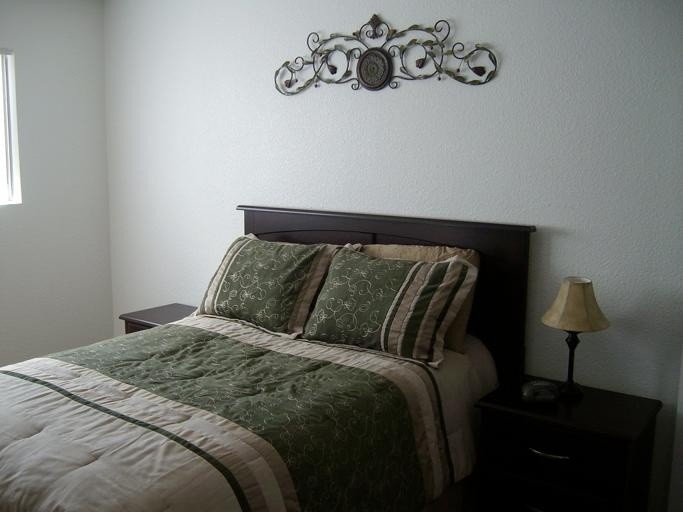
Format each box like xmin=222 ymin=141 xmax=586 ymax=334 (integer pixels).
xmin=119 ymin=302 xmax=197 ymax=336
xmin=471 ymin=374 xmax=663 ymax=511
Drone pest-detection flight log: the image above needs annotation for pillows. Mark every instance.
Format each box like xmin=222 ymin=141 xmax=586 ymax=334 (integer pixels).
xmin=196 ymin=232 xmax=481 ymax=370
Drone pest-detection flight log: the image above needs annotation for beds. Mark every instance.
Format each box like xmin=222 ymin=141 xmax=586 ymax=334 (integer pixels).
xmin=1 ymin=206 xmax=536 ymax=512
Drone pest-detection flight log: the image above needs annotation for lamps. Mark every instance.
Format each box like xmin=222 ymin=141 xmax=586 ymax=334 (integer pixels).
xmin=541 ymin=275 xmax=612 ymax=402
